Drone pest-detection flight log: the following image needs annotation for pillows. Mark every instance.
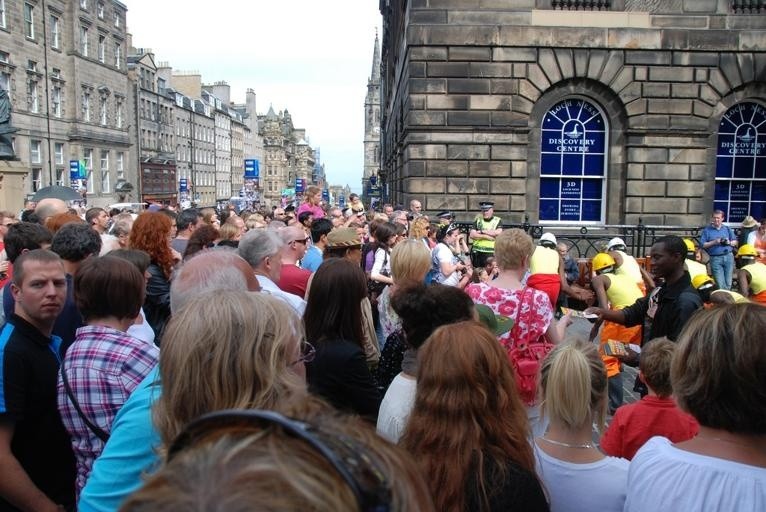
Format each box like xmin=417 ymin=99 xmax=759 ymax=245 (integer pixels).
xmin=684 ymin=238 xmax=695 ymax=252
xmin=736 ymin=244 xmax=757 ymax=257
xmin=608 ymin=237 xmax=627 ymax=252
xmin=539 ymin=232 xmax=557 ymax=247
xmin=692 ymin=275 xmax=714 ymax=289
xmin=592 ymin=252 xmax=615 ymax=271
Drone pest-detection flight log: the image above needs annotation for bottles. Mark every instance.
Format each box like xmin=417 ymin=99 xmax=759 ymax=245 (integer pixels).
xmin=323 ymin=228 xmax=365 ymax=249
xmin=479 ymin=201 xmax=493 ymax=209
xmin=742 ymin=216 xmax=755 ymax=227
xmin=436 ymin=211 xmax=450 ymax=219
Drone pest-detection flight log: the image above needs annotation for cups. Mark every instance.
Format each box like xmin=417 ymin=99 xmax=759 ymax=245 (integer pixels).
xmin=285 ymin=339 xmax=316 ymax=368
xmin=446 ymin=223 xmax=456 ymax=235
xmin=289 ymin=237 xmax=308 ymax=245
xmin=163 ymin=411 xmax=394 ymax=512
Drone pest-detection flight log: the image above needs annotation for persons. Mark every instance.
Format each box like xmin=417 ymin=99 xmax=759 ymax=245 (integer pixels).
xmin=3 ymin=185 xmax=766 ymax=512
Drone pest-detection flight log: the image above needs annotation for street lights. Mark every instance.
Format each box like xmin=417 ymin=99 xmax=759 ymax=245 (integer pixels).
xmin=505 ymin=286 xmax=554 ymax=407
xmin=367 ymin=246 xmax=388 ymax=292
